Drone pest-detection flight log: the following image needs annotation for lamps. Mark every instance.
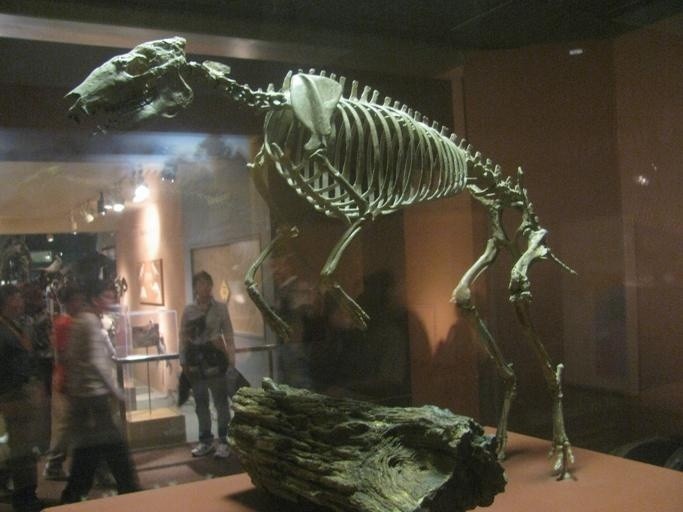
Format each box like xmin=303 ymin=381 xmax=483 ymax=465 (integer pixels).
xmin=64 ymin=188 xmax=126 ymax=232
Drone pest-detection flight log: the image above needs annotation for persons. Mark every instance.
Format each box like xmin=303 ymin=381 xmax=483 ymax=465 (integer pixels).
xmin=343 ymin=266 xmax=432 ymax=409
xmin=1 ymin=239 xmax=146 ymax=510
xmin=275 ymin=276 xmax=349 ymax=396
xmin=177 ymin=269 xmax=239 ymax=459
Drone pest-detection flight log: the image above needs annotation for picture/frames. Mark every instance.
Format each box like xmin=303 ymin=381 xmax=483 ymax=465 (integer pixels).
xmin=190 ymin=234 xmax=266 ymax=341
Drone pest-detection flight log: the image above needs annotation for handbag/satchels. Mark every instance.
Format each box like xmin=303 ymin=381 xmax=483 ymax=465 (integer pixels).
xmin=224 ymin=366 xmax=254 ymax=407
xmin=177 ymin=370 xmax=190 ymax=408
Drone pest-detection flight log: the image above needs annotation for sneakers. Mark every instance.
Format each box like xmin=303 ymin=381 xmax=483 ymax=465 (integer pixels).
xmin=190 ymin=442 xmax=213 ymax=457
xmin=0 ymin=483 xmax=60 ymax=511
xmin=42 ymin=470 xmax=67 ymax=480
xmin=213 ymin=443 xmax=229 ymax=458
